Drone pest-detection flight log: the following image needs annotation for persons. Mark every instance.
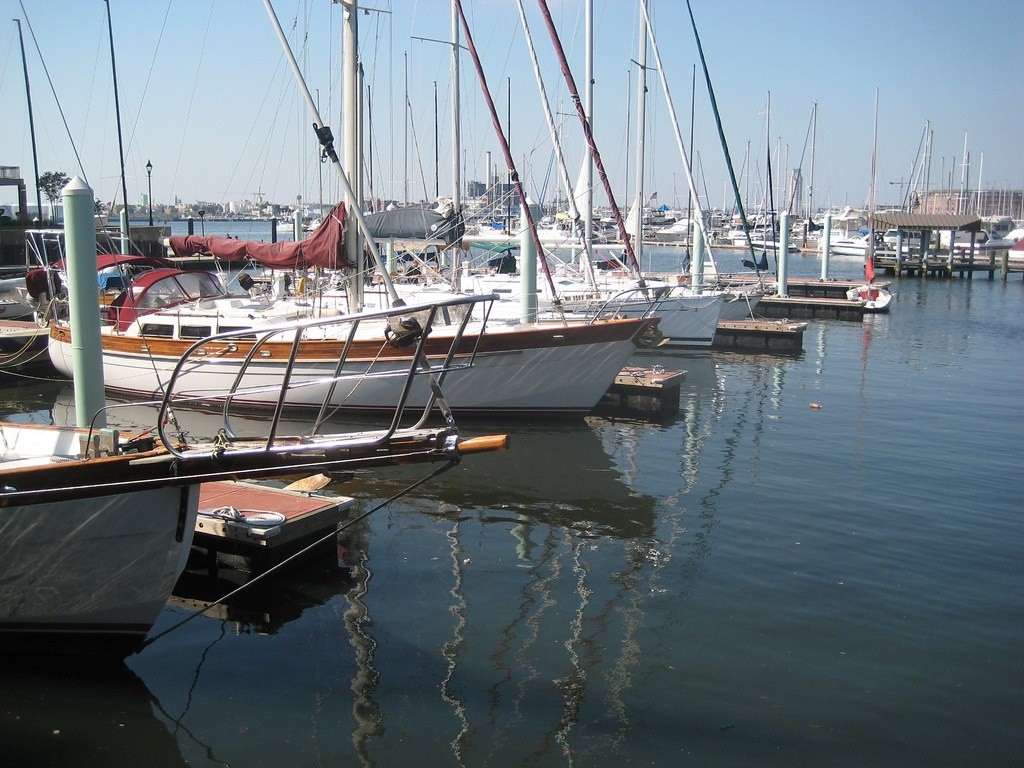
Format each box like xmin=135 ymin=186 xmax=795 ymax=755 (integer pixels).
xmin=406 ymin=261 xmax=421 ymax=284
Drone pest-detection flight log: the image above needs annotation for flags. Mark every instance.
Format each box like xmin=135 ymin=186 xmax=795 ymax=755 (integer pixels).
xmin=650 ymin=192 xmax=657 ymax=200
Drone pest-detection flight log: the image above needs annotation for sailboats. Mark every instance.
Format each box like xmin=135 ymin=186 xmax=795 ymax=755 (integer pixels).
xmin=1 ymin=1 xmax=1024 ymax=653
